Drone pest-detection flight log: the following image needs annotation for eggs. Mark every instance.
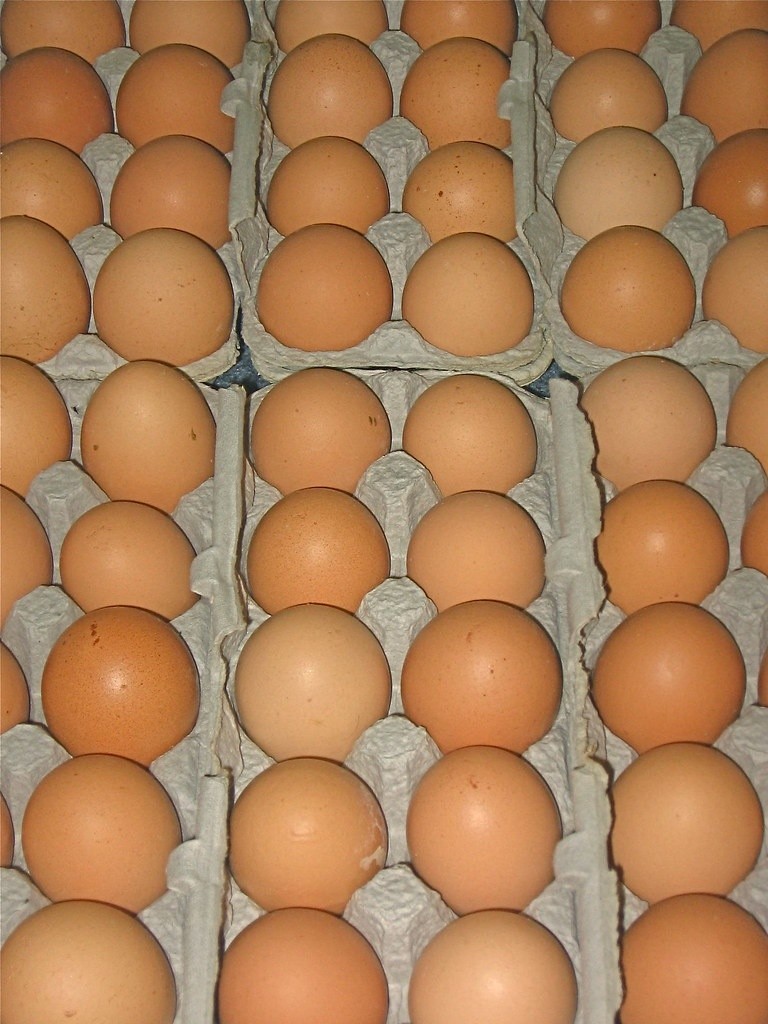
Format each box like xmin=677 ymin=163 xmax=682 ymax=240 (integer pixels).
xmin=0 ymin=0 xmax=768 ymax=1024
